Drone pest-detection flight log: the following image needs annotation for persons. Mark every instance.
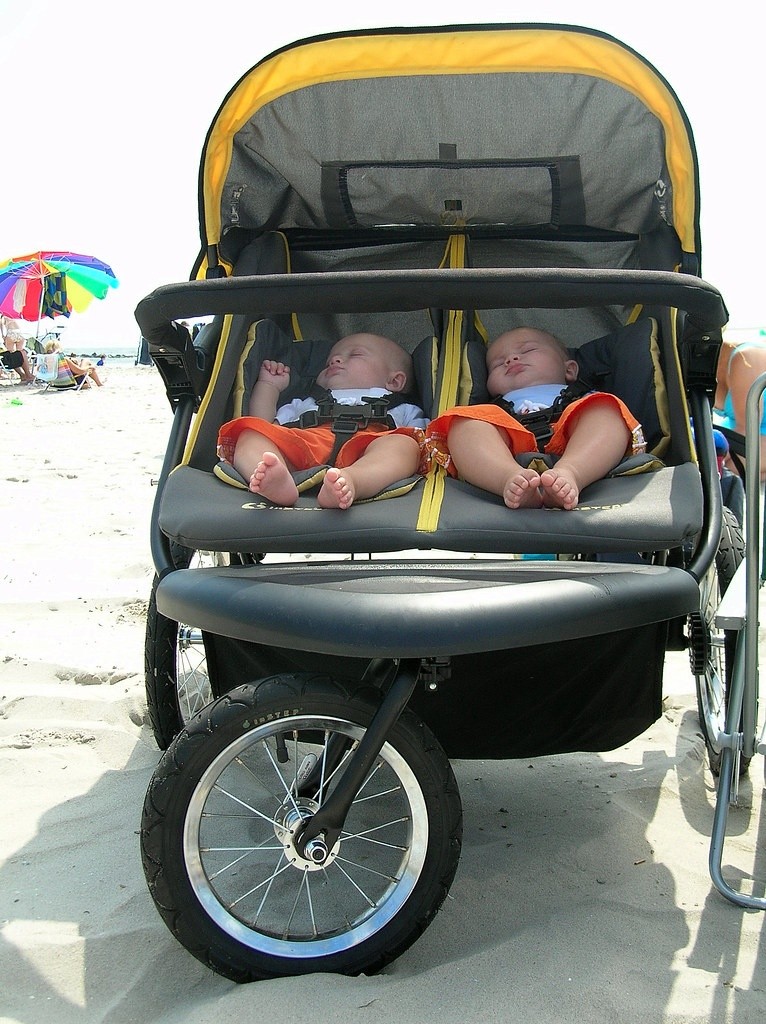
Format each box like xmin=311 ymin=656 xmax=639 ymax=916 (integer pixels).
xmin=0 ymin=316 xmax=34 ymax=380
xmin=425 ymin=327 xmax=646 ymax=511
xmin=215 ymin=332 xmax=430 ymax=510
xmin=716 ymin=339 xmax=766 ymax=483
xmin=45 ymin=340 xmax=103 ymax=388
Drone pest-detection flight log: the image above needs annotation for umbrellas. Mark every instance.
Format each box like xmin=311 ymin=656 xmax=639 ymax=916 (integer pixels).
xmin=0 ymin=250 xmax=119 ymax=338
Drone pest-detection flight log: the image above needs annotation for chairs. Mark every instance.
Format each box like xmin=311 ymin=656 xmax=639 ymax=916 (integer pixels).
xmin=0 ymin=356 xmax=15 ymax=388
xmin=28 ymin=349 xmax=91 ymax=394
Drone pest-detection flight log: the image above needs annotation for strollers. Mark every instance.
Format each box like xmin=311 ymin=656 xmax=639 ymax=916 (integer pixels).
xmin=135 ymin=22 xmax=758 ymax=987
